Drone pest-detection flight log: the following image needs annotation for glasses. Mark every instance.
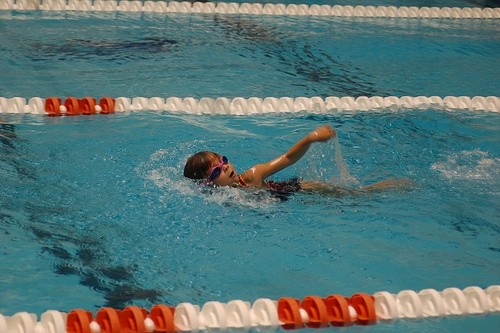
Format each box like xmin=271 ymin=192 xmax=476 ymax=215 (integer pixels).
xmin=202 ymin=153 xmax=229 ymax=185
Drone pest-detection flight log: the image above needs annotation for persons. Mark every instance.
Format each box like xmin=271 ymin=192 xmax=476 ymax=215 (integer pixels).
xmin=181 ymin=123 xmax=416 ymax=202
